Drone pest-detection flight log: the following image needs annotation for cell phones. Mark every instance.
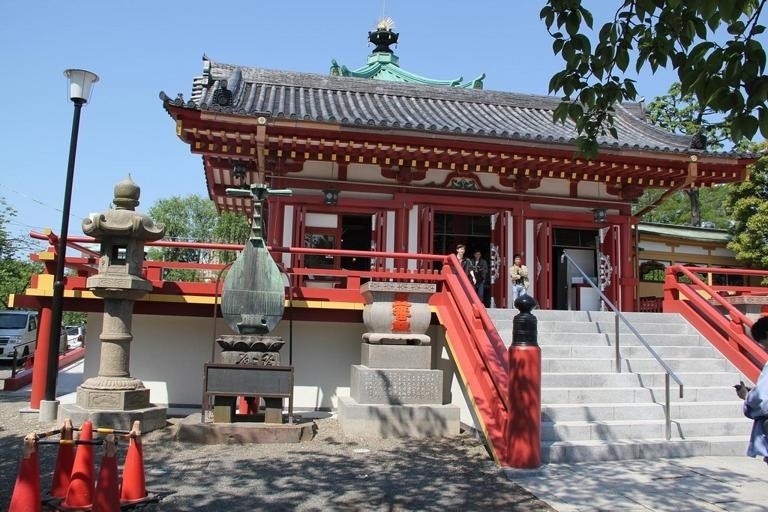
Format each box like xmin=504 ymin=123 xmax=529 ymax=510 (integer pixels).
xmin=733 ymin=384 xmax=752 ymax=392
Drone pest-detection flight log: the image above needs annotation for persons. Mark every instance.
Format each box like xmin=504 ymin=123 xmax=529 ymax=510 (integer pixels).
xmin=510 ymin=253 xmax=529 ymax=309
xmin=469 ymin=249 xmax=489 ymax=303
xmin=735 ymin=315 xmax=768 ymax=464
xmin=454 ymin=243 xmax=477 ymax=286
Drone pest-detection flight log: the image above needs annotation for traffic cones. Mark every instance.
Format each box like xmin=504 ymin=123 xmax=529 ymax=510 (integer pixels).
xmin=92 ymin=432 xmax=122 ymax=512
xmin=119 ymin=419 xmax=148 ymax=503
xmin=60 ymin=421 xmax=96 ymax=509
xmin=47 ymin=419 xmax=75 ymax=498
xmin=8 ymin=432 xmax=41 ymax=512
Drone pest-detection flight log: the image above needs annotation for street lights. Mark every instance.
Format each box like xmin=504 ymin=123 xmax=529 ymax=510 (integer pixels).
xmin=38 ymin=66 xmax=99 ymax=423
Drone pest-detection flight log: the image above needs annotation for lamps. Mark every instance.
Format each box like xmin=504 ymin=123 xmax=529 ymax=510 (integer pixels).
xmin=322 ymin=189 xmax=339 ymax=206
xmin=231 ymin=160 xmax=247 ymax=177
xmin=589 ymin=207 xmax=608 ymax=224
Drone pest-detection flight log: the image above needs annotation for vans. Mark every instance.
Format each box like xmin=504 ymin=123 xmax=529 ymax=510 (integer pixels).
xmin=0 ymin=310 xmax=66 ymax=362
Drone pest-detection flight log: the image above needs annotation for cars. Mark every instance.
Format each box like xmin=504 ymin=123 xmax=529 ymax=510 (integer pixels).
xmin=66 ymin=326 xmax=85 ymax=351
